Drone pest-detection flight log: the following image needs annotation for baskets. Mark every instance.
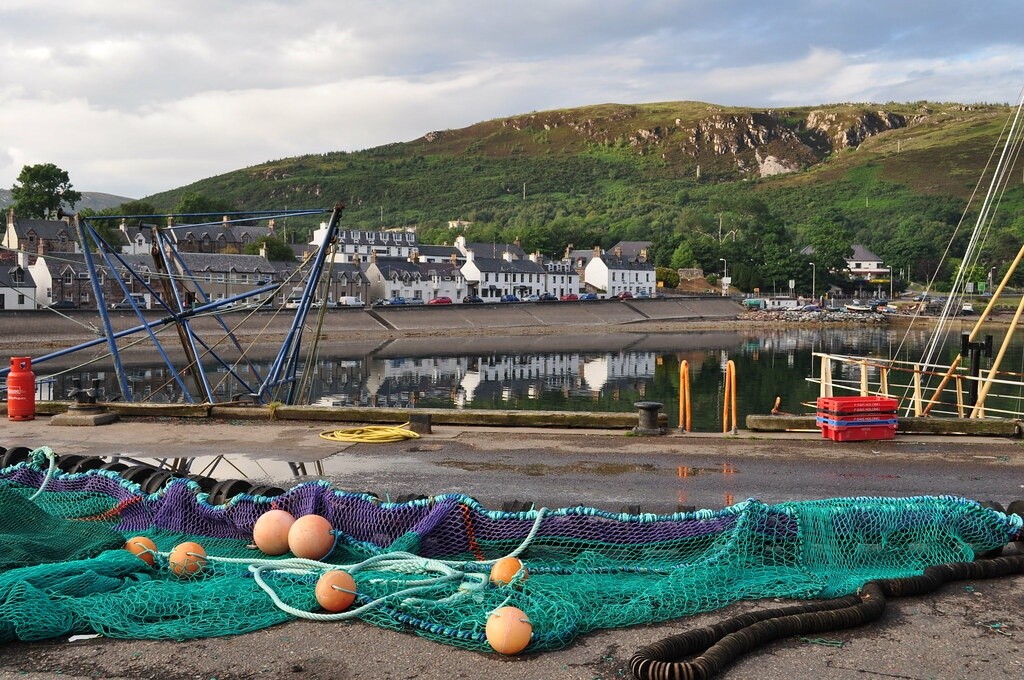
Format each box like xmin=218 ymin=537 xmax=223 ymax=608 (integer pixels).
xmin=816 ymin=396 xmax=898 ymax=441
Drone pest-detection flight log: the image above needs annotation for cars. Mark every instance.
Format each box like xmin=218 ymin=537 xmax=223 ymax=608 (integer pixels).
xmin=617 ymin=291 xmax=632 ymax=299
xmin=501 ymin=294 xmax=519 ymax=302
xmin=111 ymin=295 xmax=147 ymax=310
xmin=633 ymin=290 xmax=650 ymax=298
xmin=869 ymin=299 xmax=888 ymax=307
xmin=428 ymin=297 xmax=453 ymax=305
xmin=540 ymin=292 xmax=559 ymax=300
xmin=213 ymin=296 xmax=425 ymax=310
xmin=579 ymin=292 xmax=598 ymax=300
xmin=520 ymin=294 xmax=540 ymax=301
xmin=913 ymin=295 xmax=931 ymax=301
xmin=462 ymin=294 xmax=483 ymax=303
xmin=560 ymin=293 xmax=578 ymax=301
xmin=40 ymin=300 xmax=77 ymax=310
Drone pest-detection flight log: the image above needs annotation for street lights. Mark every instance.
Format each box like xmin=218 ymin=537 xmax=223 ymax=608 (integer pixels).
xmin=990 ymin=266 xmax=996 ymax=296
xmin=886 ymin=265 xmax=893 ymax=300
xmin=720 ymin=258 xmax=727 ymax=278
xmin=809 ymin=262 xmax=815 ymax=303
